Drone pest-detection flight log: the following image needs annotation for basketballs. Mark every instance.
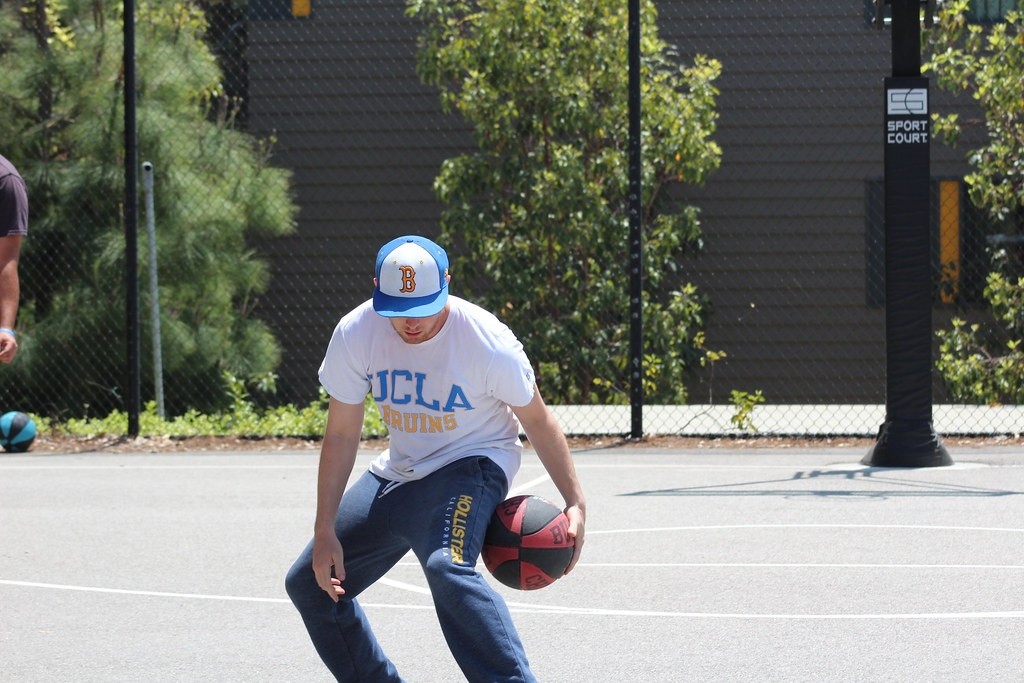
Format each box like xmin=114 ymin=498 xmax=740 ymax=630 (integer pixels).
xmin=481 ymin=495 xmax=575 ymax=590
xmin=0 ymin=410 xmax=36 ymax=453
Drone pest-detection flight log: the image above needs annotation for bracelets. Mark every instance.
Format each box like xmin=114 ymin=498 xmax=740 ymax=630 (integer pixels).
xmin=0 ymin=328 xmax=16 ymax=336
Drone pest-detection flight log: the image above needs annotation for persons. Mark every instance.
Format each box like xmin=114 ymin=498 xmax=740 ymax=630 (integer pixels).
xmin=0 ymin=153 xmax=29 ymax=363
xmin=285 ymin=235 xmax=588 ymax=683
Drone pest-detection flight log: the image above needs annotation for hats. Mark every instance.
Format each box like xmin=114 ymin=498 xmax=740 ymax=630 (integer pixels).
xmin=373 ymin=234 xmax=449 ymax=317
